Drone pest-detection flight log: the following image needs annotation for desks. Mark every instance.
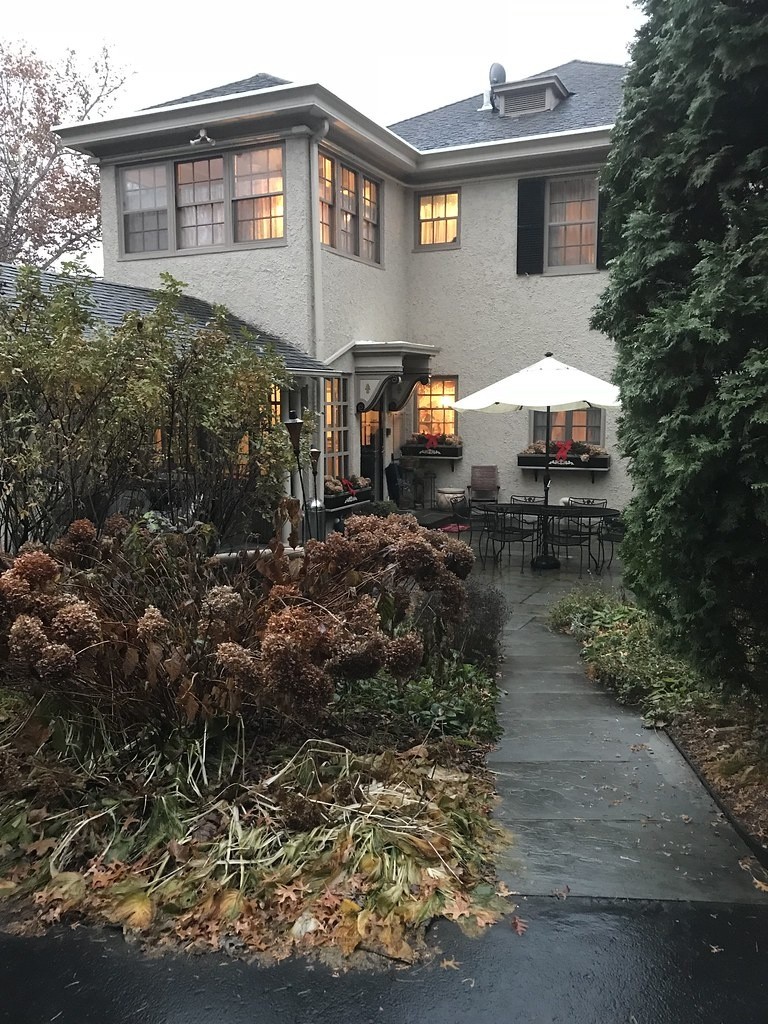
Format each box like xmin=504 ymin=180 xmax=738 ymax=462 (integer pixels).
xmin=415 ymin=473 xmax=436 ymax=509
xmin=484 ymin=504 xmax=621 ymax=573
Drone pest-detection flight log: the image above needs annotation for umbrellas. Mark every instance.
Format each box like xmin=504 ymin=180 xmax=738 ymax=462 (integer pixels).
xmin=445 ymin=351 xmax=623 ymax=568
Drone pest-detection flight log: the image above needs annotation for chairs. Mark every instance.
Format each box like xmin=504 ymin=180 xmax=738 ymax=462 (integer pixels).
xmin=449 ymin=494 xmax=626 ymax=579
xmin=467 ymin=465 xmax=500 ymax=522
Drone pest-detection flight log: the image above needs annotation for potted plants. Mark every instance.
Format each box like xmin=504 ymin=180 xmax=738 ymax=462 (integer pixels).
xmin=401 ymin=431 xmax=463 ymax=459
xmin=516 ymin=438 xmax=611 ymax=471
xmin=325 ymin=477 xmax=373 ymax=512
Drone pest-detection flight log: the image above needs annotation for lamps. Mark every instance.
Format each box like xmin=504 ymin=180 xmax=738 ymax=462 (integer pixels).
xmin=189 ymin=129 xmax=215 ymax=147
xmin=284 ymin=410 xmax=311 ymax=540
xmin=310 ymin=444 xmax=320 ymax=541
